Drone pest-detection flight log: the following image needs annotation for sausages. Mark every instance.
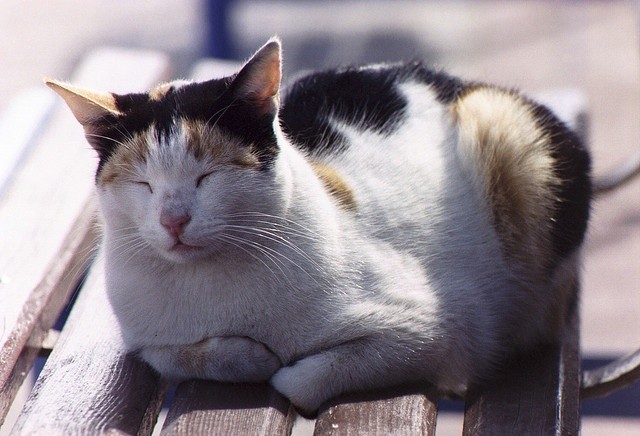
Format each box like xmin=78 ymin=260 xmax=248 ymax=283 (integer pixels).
xmin=43 ymin=36 xmax=594 ymax=413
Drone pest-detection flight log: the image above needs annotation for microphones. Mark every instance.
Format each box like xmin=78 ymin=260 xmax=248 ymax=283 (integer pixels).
xmin=0 ymin=47 xmax=587 ymax=435
